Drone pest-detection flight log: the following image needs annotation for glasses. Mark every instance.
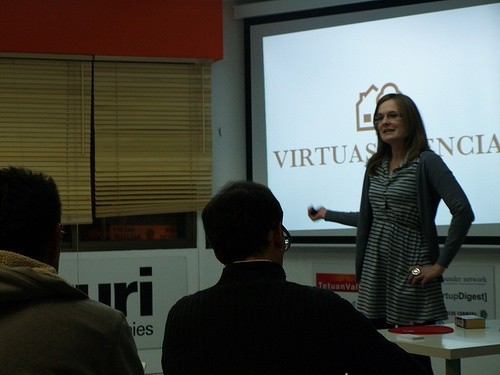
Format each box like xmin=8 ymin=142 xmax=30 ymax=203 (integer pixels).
xmin=375 ymin=113 xmax=402 ymax=121
xmin=281 ymin=224 xmax=292 ymax=251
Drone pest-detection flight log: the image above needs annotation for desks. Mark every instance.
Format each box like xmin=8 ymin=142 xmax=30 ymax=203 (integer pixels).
xmin=375 ymin=320 xmax=500 ymax=375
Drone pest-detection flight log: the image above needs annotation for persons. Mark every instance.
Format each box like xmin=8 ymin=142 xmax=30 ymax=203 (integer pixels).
xmin=0 ymin=165 xmax=146 ymax=375
xmin=309 ymin=93 xmax=474 ymax=375
xmin=160 ymin=179 xmax=423 ymax=375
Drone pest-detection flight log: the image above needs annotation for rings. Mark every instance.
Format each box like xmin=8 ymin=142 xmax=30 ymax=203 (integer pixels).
xmin=411 ymin=267 xmax=421 ymax=276
